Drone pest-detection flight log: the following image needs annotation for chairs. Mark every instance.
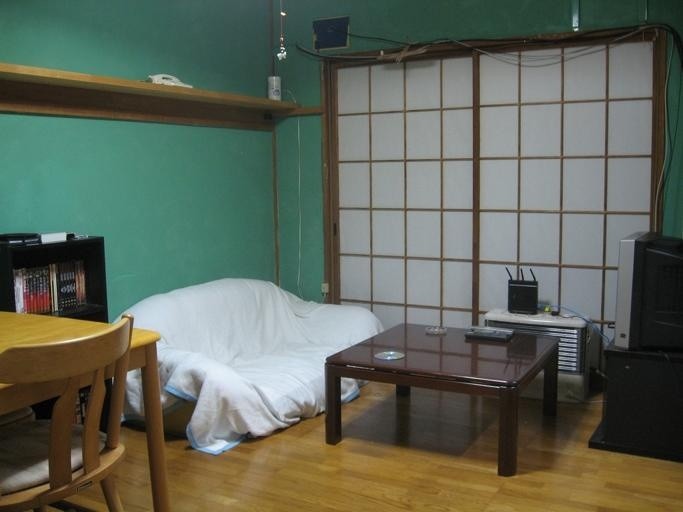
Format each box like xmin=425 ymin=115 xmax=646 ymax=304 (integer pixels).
xmin=0 ymin=313 xmax=134 ymax=512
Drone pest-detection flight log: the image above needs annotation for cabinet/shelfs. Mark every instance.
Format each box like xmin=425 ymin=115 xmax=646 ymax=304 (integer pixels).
xmin=0 ymin=233 xmax=113 ymax=437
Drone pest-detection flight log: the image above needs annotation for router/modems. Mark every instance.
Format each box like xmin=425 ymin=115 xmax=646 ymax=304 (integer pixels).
xmin=503 ymin=266 xmax=540 ymax=315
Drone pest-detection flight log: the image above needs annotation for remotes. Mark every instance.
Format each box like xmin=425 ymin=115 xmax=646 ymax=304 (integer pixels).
xmin=464 ymin=330 xmax=510 ymax=344
xmin=466 ymin=325 xmax=516 ymax=336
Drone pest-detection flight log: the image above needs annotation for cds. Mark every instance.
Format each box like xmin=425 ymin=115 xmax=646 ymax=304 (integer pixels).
xmin=374 ymin=350 xmax=406 ymax=360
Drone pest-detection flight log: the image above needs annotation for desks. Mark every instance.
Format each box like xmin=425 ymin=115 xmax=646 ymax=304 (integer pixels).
xmin=0 ymin=309 xmax=174 ymax=512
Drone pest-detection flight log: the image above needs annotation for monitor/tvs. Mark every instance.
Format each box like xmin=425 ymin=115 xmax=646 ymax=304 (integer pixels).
xmin=613 ymin=230 xmax=683 ymax=351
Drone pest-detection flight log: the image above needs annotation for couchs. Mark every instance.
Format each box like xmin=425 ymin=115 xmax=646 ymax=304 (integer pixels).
xmin=110 ymin=275 xmax=380 ymax=444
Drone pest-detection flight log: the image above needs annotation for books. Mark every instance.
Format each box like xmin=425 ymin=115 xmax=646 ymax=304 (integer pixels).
xmin=71 ymin=387 xmax=91 ymax=424
xmin=12 ymin=259 xmax=86 ymax=316
